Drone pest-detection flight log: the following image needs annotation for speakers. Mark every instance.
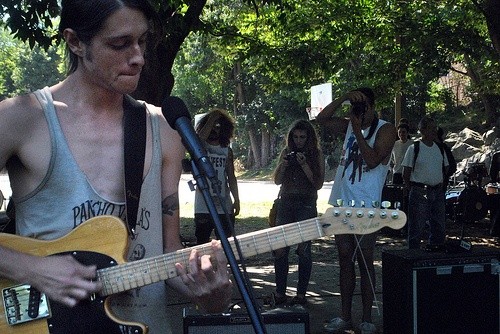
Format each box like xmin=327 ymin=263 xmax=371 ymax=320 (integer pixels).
xmin=382 ymin=244 xmax=500 ymax=334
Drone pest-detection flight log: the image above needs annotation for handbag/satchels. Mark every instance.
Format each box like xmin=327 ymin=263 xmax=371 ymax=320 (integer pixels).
xmin=268 ymin=198 xmax=280 ymax=227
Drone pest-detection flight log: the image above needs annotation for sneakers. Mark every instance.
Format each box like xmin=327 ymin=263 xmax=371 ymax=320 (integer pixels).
xmin=358 ymin=322 xmax=377 ymax=334
xmin=322 ymin=316 xmax=354 ymax=332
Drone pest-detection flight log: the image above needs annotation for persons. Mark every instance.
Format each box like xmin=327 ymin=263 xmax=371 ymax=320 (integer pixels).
xmin=191 ymin=108 xmax=240 ymax=245
xmin=398 ymin=118 xmax=413 ymax=140
xmin=268 ymin=118 xmax=326 ymax=306
xmin=424 ymin=126 xmax=458 ymax=252
xmin=0 ymin=0 xmax=232 ymax=334
xmin=316 ymin=87 xmax=397 ymax=334
xmin=388 ymin=123 xmax=415 ymax=185
xmin=401 ymin=117 xmax=449 ymax=250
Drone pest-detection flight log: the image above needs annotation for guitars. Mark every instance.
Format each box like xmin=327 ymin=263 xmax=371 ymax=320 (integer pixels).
xmin=0 ymin=199 xmax=407 ymax=334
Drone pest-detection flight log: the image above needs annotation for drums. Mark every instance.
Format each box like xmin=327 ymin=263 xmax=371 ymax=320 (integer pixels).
xmin=485 ymin=182 xmax=500 ymax=195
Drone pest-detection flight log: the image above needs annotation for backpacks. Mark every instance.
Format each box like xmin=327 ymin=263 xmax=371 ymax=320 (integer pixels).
xmin=439 ymin=143 xmax=456 ymax=177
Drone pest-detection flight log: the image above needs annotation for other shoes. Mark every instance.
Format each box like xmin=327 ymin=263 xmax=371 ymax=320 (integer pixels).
xmin=291 ymin=296 xmax=307 ymax=304
xmin=263 ymin=293 xmax=287 ymax=305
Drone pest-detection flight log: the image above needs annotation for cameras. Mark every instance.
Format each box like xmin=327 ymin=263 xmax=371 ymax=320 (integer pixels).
xmin=350 ymin=96 xmax=365 ymax=124
xmin=283 ymin=145 xmax=305 ymax=159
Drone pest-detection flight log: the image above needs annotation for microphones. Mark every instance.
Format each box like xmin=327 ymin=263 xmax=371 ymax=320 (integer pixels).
xmin=161 ymin=96 xmax=217 ymax=185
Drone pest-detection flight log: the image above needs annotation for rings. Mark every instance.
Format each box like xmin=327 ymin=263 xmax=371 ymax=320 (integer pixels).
xmin=300 ymin=157 xmax=304 ymax=160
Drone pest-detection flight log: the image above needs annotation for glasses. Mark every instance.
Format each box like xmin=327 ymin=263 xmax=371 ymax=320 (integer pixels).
xmin=213 ymin=123 xmax=223 ymax=128
